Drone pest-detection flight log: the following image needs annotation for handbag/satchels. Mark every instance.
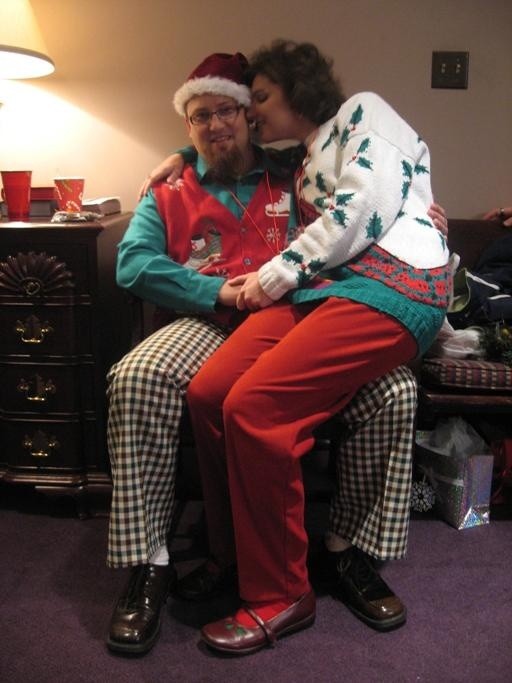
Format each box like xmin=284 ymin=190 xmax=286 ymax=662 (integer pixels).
xmin=414 ymin=428 xmax=495 ymax=531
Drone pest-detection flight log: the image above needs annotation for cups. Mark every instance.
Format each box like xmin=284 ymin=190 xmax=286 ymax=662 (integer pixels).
xmin=0 ymin=170 xmax=32 ymax=222
xmin=54 ymin=178 xmax=85 ymax=212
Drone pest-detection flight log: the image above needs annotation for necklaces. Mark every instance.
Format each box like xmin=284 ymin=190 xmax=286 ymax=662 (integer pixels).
xmin=207 ymin=169 xmax=280 ymax=256
xmin=294 ymin=127 xmax=318 ymax=231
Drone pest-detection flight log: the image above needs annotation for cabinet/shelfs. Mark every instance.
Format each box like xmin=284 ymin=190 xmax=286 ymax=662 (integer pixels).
xmin=0 ymin=210 xmax=136 ymax=520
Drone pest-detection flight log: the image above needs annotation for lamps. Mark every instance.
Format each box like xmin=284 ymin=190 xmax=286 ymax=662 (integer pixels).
xmin=0 ymin=45 xmax=55 ymax=81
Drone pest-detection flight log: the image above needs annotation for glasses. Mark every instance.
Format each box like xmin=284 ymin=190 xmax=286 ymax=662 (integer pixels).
xmin=186 ymin=101 xmax=249 ymax=126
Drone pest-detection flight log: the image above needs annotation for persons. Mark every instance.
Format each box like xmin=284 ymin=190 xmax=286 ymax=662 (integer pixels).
xmin=481 ymin=206 xmax=511 ymax=228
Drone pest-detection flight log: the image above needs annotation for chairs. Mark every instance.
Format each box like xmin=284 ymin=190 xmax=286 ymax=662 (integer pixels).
xmin=419 ymin=217 xmax=512 ymax=418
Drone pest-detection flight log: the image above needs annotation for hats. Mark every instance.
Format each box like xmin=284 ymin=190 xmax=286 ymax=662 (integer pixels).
xmin=171 ymin=51 xmax=253 ymax=117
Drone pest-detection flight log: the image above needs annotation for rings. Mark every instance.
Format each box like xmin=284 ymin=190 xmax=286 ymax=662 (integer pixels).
xmin=500 ymin=207 xmax=504 ymax=215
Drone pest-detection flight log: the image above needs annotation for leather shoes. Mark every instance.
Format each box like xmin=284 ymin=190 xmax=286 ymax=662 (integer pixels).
xmin=323 ymin=545 xmax=408 ymax=631
xmin=175 ymin=558 xmax=238 ymax=599
xmin=106 ymin=564 xmax=177 ymax=654
xmin=201 ymin=588 xmax=316 ymax=656
